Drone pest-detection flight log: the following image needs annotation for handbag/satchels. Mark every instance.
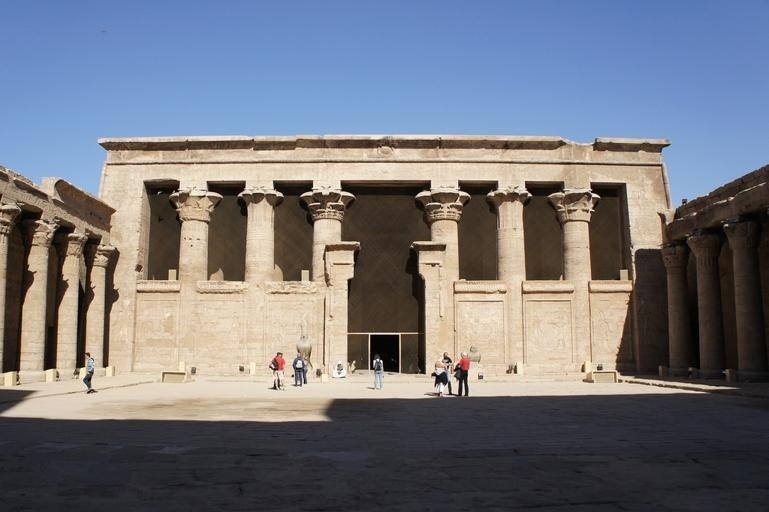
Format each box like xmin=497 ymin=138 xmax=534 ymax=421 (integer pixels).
xmin=269 ymin=359 xmax=279 ymax=370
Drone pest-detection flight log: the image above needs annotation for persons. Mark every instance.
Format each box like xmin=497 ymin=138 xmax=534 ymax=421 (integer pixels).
xmin=373 ymin=353 xmax=384 ymax=390
xmin=434 ymin=355 xmax=447 ymax=397
xmin=271 ymin=351 xmax=284 ymax=390
xmin=348 ymin=359 xmax=353 ymax=375
xmin=332 ymin=360 xmax=346 ymax=378
xmin=273 ymin=353 xmax=284 ymax=389
xmin=456 ymin=351 xmax=470 ymax=396
xmin=72 ymin=368 xmax=80 ymax=379
xmin=302 ymin=357 xmax=308 ymax=384
xmin=438 ymin=352 xmax=454 ymax=396
xmin=82 ymin=352 xmax=95 ymax=393
xmin=351 ymin=359 xmax=357 ymax=375
xmin=293 ymin=352 xmax=304 ymax=387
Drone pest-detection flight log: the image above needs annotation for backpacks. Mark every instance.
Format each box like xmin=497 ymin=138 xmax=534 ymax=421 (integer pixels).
xmin=375 ymin=360 xmax=382 ymax=372
xmin=296 ymin=360 xmax=303 ymax=369
